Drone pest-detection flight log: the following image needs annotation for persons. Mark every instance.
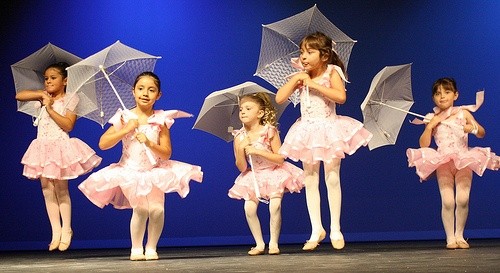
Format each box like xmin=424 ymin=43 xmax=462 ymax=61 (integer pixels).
xmin=406 ymin=77 xmax=500 ymax=249
xmin=228 ymin=94 xmax=306 ymax=255
xmin=16 ymin=63 xmax=102 ymax=251
xmin=78 ymin=71 xmax=203 ymax=260
xmin=275 ymin=31 xmax=373 ymax=250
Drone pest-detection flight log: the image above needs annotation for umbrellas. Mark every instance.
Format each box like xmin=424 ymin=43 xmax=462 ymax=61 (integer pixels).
xmin=192 ymin=81 xmax=291 ymax=198
xmin=10 ymin=41 xmax=98 ymax=126
xmin=253 ymin=4 xmax=358 ymax=108
xmin=360 ymin=64 xmax=464 ymax=150
xmin=62 ymin=39 xmax=161 ymax=166
